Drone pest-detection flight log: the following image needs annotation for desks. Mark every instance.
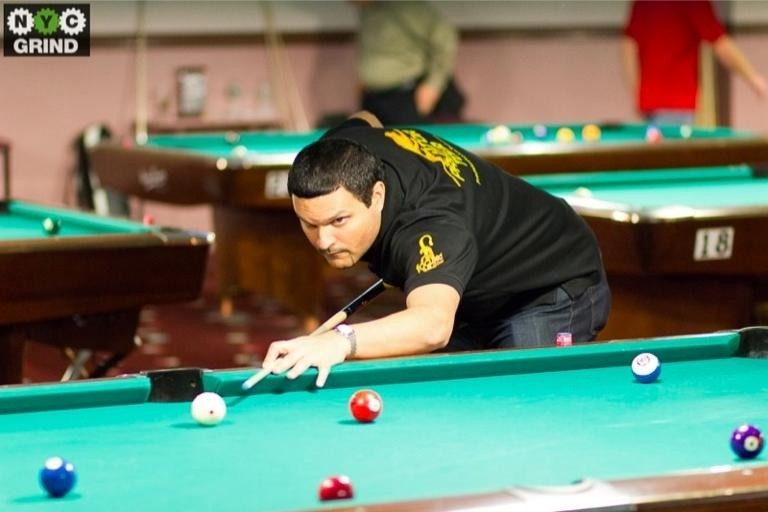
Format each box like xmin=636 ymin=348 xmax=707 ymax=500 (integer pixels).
xmin=0 ymin=122 xmax=768 ymax=512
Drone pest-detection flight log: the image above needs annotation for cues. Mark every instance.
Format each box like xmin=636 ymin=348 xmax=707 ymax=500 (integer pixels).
xmin=242 ymin=279 xmax=389 ymax=391
xmin=260 ymin=0 xmax=311 ymax=130
xmin=135 ymin=0 xmax=146 ymax=222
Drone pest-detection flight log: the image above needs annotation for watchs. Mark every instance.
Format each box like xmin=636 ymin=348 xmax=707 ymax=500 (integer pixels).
xmin=332 ymin=324 xmax=357 ymax=361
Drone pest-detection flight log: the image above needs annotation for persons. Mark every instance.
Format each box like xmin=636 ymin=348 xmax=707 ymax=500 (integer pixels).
xmin=261 ymin=109 xmax=610 ymax=387
xmin=619 ymin=0 xmax=768 ymax=124
xmin=346 ymin=0 xmax=468 ymax=130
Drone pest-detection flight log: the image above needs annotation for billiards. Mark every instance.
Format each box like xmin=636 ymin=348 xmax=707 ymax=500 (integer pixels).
xmin=485 ymin=123 xmax=691 ymax=144
